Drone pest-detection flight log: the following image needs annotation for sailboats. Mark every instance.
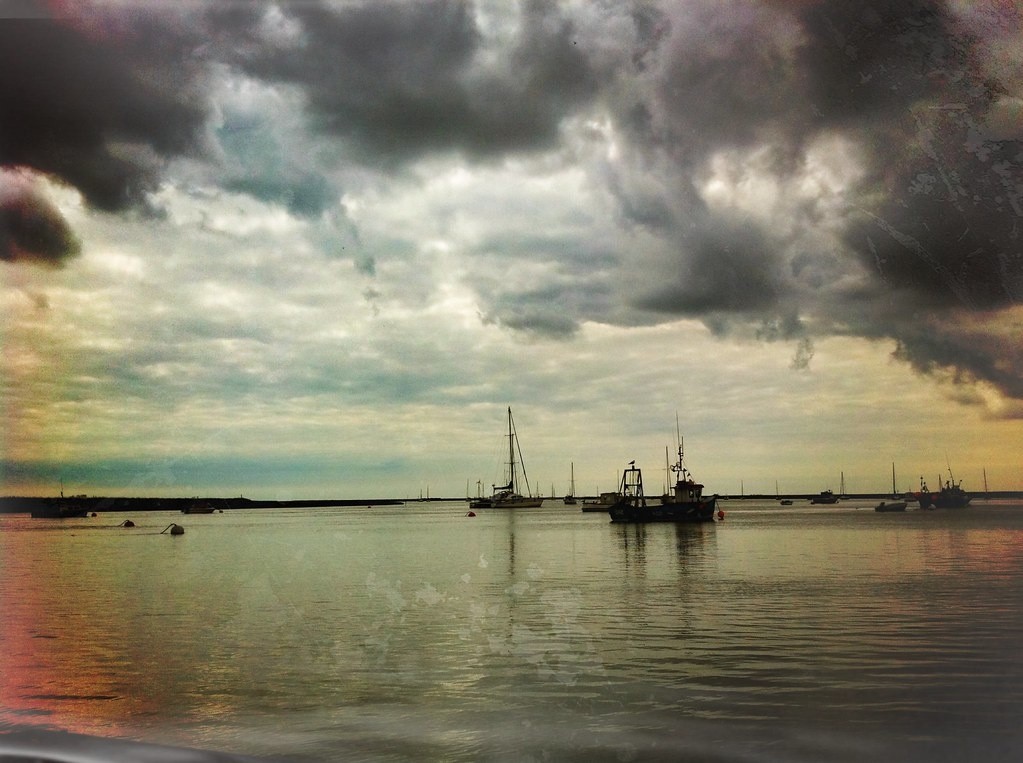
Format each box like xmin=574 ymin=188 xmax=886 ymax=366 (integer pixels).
xmin=656 ymin=443 xmax=677 ymax=505
xmin=606 ymin=414 xmax=719 ymax=524
xmin=581 ymin=470 xmax=625 ymax=512
xmin=914 ymin=453 xmax=974 ymax=509
xmin=418 ymin=486 xmax=431 ymax=502
xmin=562 ymin=462 xmax=578 ymax=505
xmin=470 ymin=407 xmax=544 ymax=510
xmin=891 ymin=462 xmax=901 ymax=500
xmin=838 ymin=471 xmax=850 ymax=501
xmin=775 ymin=480 xmax=782 ymax=500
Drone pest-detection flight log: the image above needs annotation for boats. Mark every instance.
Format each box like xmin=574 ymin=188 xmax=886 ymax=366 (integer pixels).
xmin=30 ymin=492 xmax=95 ymax=520
xmin=780 ymin=500 xmax=793 ymax=506
xmin=810 ymin=489 xmax=840 ymax=505
xmin=902 ymin=481 xmax=917 ymax=502
xmin=874 ymin=501 xmax=908 ymax=513
xmin=983 ymin=469 xmax=992 ymax=500
xmin=181 ymin=497 xmax=215 ymax=514
